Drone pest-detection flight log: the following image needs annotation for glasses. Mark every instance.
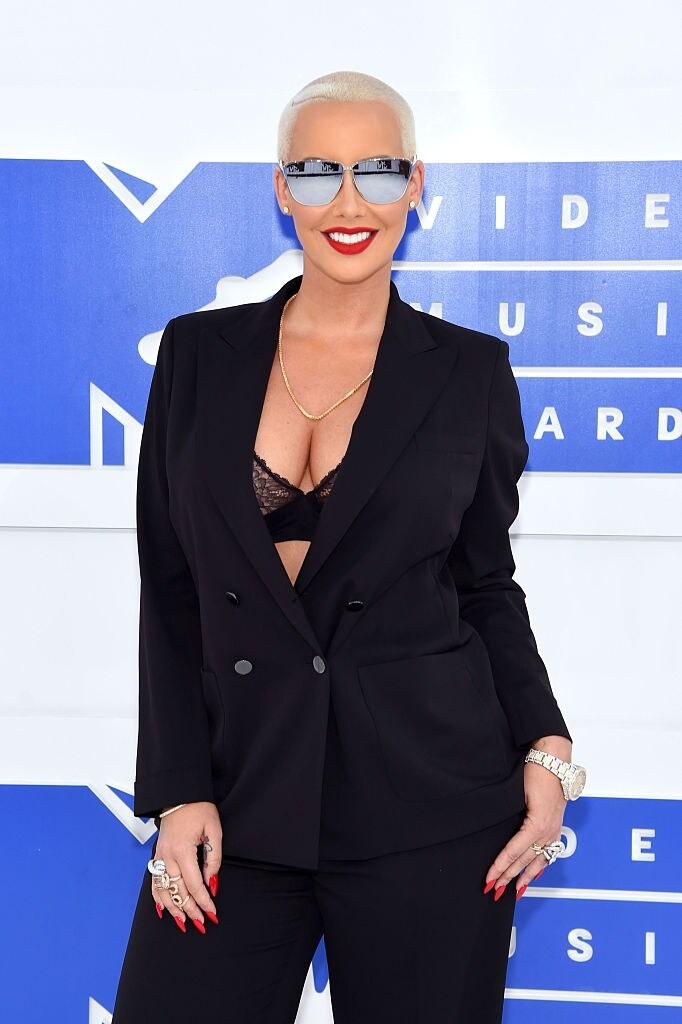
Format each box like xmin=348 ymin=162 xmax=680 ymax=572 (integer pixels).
xmin=278 ymin=155 xmax=417 ymax=206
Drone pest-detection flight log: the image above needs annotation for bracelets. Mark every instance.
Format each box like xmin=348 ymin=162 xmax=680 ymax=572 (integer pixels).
xmin=158 ymin=804 xmax=187 ymax=818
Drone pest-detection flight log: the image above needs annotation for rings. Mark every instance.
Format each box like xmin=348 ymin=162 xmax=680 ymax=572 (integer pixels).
xmin=530 ymin=843 xmax=544 ymax=855
xmin=544 ymin=841 xmax=565 ymax=865
xmin=147 ymin=859 xmax=190 ymax=911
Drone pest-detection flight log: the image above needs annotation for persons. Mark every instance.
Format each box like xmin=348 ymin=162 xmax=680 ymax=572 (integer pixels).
xmin=112 ymin=70 xmax=574 ymax=1024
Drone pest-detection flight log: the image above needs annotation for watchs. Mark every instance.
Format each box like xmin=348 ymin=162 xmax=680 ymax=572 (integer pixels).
xmin=525 ymin=749 xmax=587 ymax=801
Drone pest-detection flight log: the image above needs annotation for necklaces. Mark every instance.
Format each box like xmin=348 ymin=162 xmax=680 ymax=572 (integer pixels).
xmin=278 ymin=294 xmax=374 ymax=421
